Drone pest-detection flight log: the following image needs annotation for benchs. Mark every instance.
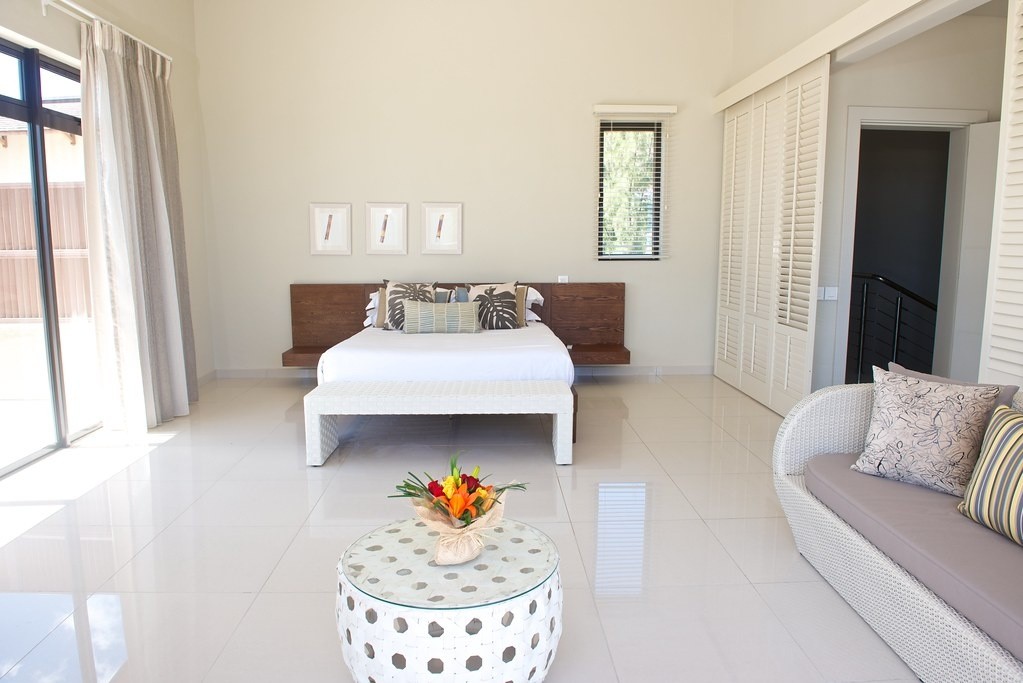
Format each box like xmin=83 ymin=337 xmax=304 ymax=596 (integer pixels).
xmin=773 ymin=383 xmax=1023 ymax=683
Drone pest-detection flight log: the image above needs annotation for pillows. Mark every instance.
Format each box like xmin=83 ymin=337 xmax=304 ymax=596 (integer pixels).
xmin=958 ymin=404 xmax=1023 ymax=545
xmin=888 ymin=362 xmax=1020 ymax=429
xmin=363 ymin=279 xmax=545 ymax=334
xmin=851 ymin=365 xmax=1003 ymax=498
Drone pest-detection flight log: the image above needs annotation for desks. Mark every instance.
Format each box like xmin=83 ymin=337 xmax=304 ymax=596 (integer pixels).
xmin=337 ymin=519 xmax=563 ymax=683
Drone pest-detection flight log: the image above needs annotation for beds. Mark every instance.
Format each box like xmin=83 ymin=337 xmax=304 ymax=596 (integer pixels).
xmin=282 ymin=282 xmax=630 ymax=464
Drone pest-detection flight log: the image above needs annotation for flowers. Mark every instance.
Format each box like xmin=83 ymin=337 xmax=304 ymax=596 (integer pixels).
xmin=386 ymin=451 xmax=528 ymax=565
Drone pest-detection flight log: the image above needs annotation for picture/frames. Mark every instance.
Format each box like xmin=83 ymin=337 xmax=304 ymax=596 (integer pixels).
xmin=310 ymin=203 xmax=352 ymax=257
xmin=420 ymin=202 xmax=463 ymax=255
xmin=364 ymin=202 xmax=408 ymax=255
